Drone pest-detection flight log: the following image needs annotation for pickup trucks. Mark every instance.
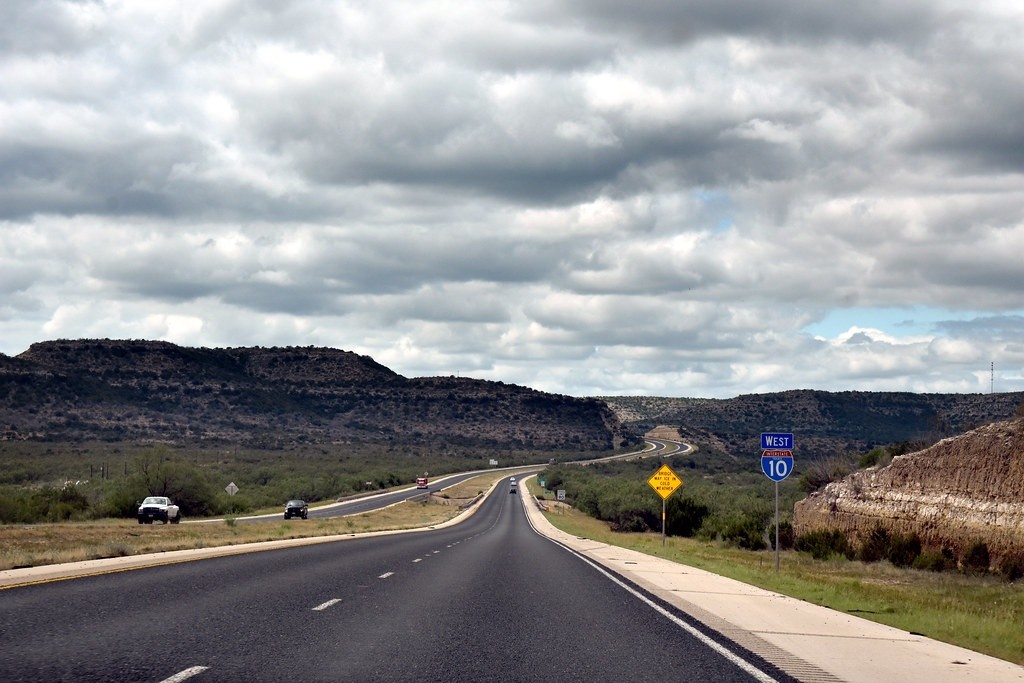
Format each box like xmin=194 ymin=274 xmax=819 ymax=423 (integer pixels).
xmin=136 ymin=496 xmax=181 ymax=527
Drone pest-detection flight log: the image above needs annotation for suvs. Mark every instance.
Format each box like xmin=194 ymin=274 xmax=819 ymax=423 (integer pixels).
xmin=283 ymin=500 xmax=309 ymax=520
xmin=417 ymin=478 xmax=428 ymax=490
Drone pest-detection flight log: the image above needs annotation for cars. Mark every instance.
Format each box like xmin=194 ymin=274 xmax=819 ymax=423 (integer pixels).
xmin=509 ymin=488 xmax=516 ymax=494
xmin=510 ymin=477 xmax=515 ymax=481
xmin=511 ymin=481 xmax=517 ymax=486
